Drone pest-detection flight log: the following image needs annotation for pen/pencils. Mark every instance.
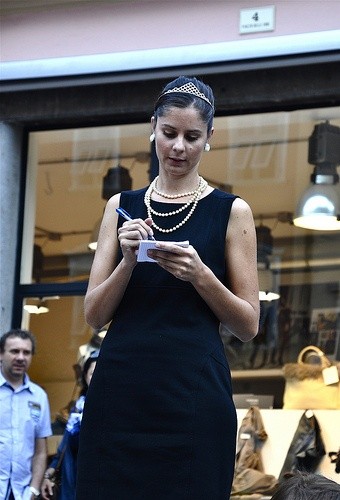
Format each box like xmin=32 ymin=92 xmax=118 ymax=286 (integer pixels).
xmin=116 ymin=207 xmax=155 ymax=239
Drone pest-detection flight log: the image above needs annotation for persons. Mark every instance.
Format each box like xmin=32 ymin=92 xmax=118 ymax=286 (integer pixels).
xmin=75 ymin=76 xmax=260 ymax=500
xmin=0 ymin=331 xmax=53 ymax=500
xmin=38 ymin=351 xmax=99 ymax=500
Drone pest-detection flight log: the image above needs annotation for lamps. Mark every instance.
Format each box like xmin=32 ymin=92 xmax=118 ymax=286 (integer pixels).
xmin=293 ymin=122 xmax=340 ymax=232
xmin=88 ymin=157 xmax=133 ymax=250
xmin=254 ymin=214 xmax=280 ymax=300
xmin=24 ymin=244 xmax=50 ymax=313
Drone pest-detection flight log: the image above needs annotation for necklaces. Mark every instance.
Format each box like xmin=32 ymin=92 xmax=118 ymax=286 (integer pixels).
xmin=144 ymin=176 xmax=209 ymax=232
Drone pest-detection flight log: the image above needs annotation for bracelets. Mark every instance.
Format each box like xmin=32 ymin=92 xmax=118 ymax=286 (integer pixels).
xmin=43 ymin=473 xmax=52 ymax=480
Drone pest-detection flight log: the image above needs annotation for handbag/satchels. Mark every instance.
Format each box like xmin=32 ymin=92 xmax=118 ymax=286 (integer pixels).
xmin=281 ymin=346 xmax=340 ymax=409
xmin=35 ymin=468 xmax=62 ymax=500
